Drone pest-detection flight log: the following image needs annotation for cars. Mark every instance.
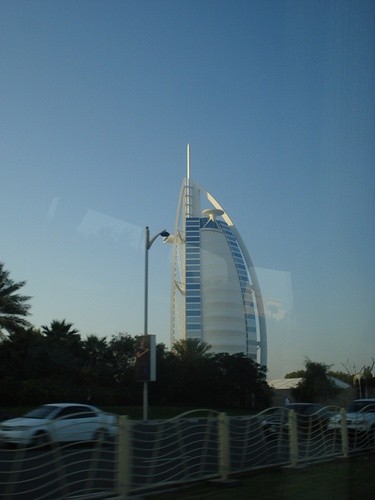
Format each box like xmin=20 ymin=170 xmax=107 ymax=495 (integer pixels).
xmin=326 ymin=398 xmax=375 ymax=442
xmin=257 ymin=402 xmax=328 ymax=441
xmin=0 ymin=402 xmax=121 ymax=448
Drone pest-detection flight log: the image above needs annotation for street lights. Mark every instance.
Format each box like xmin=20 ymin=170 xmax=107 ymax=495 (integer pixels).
xmin=143 ymin=226 xmax=170 ymax=425
